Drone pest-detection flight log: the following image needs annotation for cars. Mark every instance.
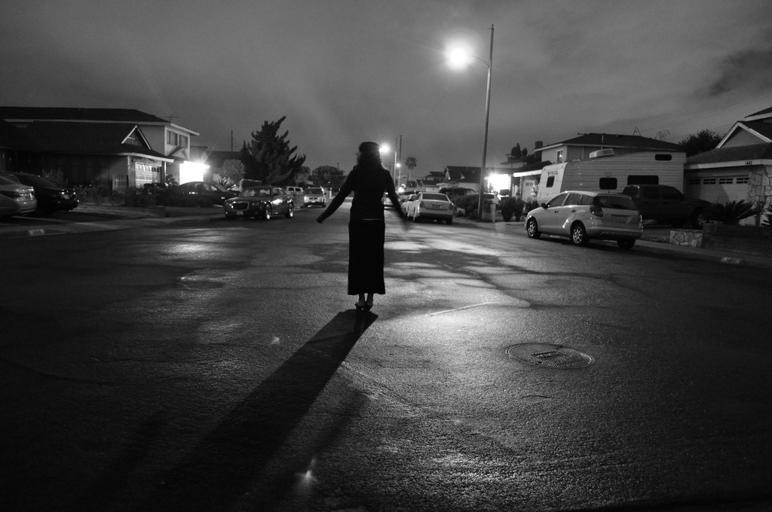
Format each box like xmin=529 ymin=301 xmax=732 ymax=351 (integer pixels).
xmin=143 ymin=179 xmax=325 ymax=225
xmin=0 ymin=170 xmax=80 ymax=219
xmin=382 ymin=181 xmax=498 ymax=224
xmin=524 ymin=189 xmax=644 ymax=248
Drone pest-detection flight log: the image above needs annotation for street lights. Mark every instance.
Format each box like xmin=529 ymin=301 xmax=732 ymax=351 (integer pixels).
xmin=395 ymin=163 xmax=403 ymax=186
xmin=446 ymin=23 xmax=497 ymax=219
xmin=381 ymin=144 xmax=398 ymax=187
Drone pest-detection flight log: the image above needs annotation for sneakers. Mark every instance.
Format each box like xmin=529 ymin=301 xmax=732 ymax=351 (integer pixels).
xmin=354 ymin=299 xmax=373 ymax=309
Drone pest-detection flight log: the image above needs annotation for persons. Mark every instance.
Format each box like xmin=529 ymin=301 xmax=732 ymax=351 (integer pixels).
xmin=315 ymin=142 xmax=407 ymax=310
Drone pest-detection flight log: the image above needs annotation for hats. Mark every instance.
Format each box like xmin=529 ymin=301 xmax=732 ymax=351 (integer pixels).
xmin=359 ymin=141 xmax=381 ymax=153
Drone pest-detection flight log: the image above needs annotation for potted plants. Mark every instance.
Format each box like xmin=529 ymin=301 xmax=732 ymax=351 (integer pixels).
xmin=700 ymin=201 xmax=759 ymax=236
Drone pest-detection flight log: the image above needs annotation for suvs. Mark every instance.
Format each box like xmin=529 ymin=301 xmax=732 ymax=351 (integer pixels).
xmin=624 ymin=184 xmax=713 ymax=226
